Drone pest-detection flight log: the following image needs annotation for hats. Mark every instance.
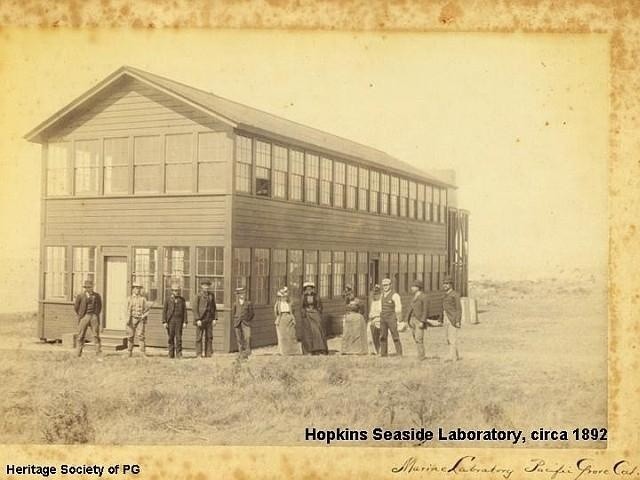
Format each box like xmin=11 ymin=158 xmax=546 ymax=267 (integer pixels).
xmin=412 ymin=279 xmax=423 ymax=288
xmin=202 ymin=280 xmax=211 ymax=285
xmin=277 ymin=287 xmax=288 ymax=296
xmin=303 ymin=282 xmax=315 ymax=289
xmin=234 ymin=288 xmax=246 ymax=294
xmin=169 ymin=284 xmax=180 ymax=290
xmin=82 ymin=280 xmax=95 ymax=288
xmin=131 ymin=283 xmax=143 ymax=287
xmin=382 ymin=279 xmax=391 ymax=285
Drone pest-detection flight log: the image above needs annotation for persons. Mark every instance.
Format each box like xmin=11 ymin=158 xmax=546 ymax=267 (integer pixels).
xmin=341 ymin=284 xmax=368 ymax=355
xmin=256 ymin=179 xmax=268 ymax=194
xmin=377 ymin=277 xmax=404 ymax=357
xmin=192 ymin=278 xmax=218 ymax=359
xmin=300 ymin=281 xmax=328 ymax=355
xmin=124 ymin=281 xmax=151 ymax=360
xmin=437 ymin=276 xmax=463 ymax=363
xmin=369 ymin=283 xmax=383 ymax=355
xmin=407 ymin=280 xmax=431 ymax=361
xmin=161 ymin=283 xmax=188 ymax=360
xmin=272 ymin=287 xmax=300 ymax=354
xmin=230 ymin=287 xmax=255 ymax=361
xmin=73 ymin=280 xmax=103 ymax=359
